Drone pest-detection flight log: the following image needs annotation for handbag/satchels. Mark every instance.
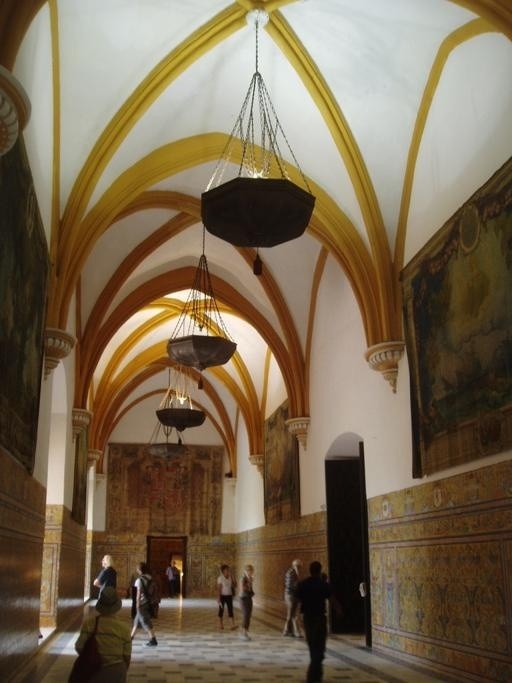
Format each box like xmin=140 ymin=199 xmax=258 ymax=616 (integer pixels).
xmin=68 ymin=637 xmax=99 ymax=683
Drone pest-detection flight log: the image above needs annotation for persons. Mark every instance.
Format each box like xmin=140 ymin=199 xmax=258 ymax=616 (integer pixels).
xmin=127 ymin=560 xmax=158 ymax=646
xmin=279 ymin=557 xmax=305 ymax=639
xmin=75 ymin=585 xmax=133 ymax=682
xmin=164 ymin=563 xmax=174 ymax=597
xmin=171 ymin=560 xmax=180 ymax=595
xmin=237 ymin=564 xmax=256 ymax=641
xmin=292 ymin=560 xmax=335 ymax=682
xmin=93 ymin=553 xmax=118 ymax=602
xmin=214 ymin=563 xmax=239 ymax=631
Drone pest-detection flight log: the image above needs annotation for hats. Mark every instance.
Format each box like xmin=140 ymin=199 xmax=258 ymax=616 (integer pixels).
xmin=96 ymin=587 xmax=122 ymax=617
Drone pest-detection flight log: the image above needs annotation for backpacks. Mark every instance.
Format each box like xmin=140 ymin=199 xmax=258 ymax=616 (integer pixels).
xmin=139 ymin=576 xmax=159 ymax=609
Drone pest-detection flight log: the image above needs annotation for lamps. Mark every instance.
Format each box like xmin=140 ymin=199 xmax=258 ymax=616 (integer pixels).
xmin=165 ymin=256 xmax=240 ymax=387
xmin=154 ymin=361 xmax=207 ymax=447
xmin=196 ymin=73 xmax=319 ymax=263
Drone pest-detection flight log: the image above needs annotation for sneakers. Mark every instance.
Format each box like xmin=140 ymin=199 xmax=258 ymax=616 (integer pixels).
xmin=293 ymin=631 xmax=303 ymax=637
xmin=283 ymin=631 xmax=293 ymax=636
xmin=230 ymin=625 xmax=238 ymax=631
xmin=239 ymin=636 xmax=250 ymax=641
xmin=147 ymin=641 xmax=158 ymax=646
